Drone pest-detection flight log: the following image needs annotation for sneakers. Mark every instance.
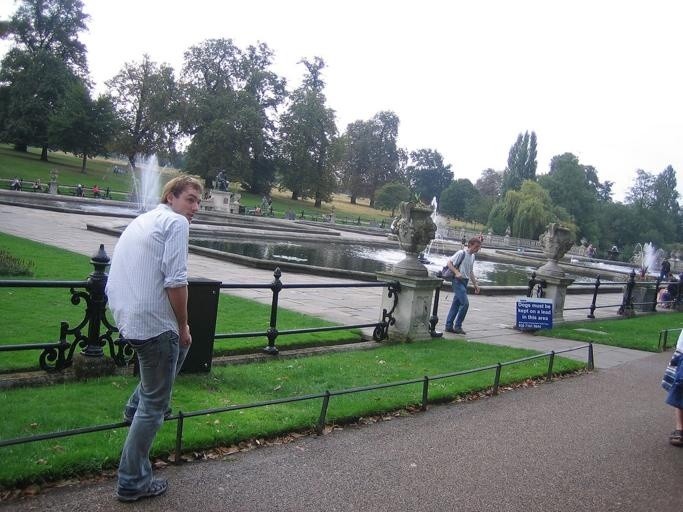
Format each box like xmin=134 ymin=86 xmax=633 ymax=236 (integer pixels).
xmin=116 ymin=478 xmax=168 ymax=502
xmin=670 ymin=430 xmax=683 ymax=445
xmin=123 ymin=407 xmax=172 ymax=422
xmin=445 ymin=326 xmax=465 ymax=334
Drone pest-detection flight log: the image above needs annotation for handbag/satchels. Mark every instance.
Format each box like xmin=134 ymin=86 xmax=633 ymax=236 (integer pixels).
xmin=441 ymin=263 xmax=459 ymax=281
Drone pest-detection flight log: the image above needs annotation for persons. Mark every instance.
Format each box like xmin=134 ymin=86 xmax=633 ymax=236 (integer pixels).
xmin=659 ymin=327 xmax=682 ymax=448
xmin=92 ymin=184 xmax=101 ymax=199
xmin=585 ymin=244 xmax=593 ymax=258
xmin=657 ymin=257 xmax=669 ymax=283
xmin=11 ymin=177 xmax=22 ymax=192
xmin=461 ymin=235 xmax=465 ymax=248
xmin=105 ymin=173 xmax=205 ymax=504
xmin=251 ymin=195 xmax=296 ymax=220
xmin=32 ymin=178 xmax=43 ymax=191
xmin=478 ymin=231 xmax=484 ymax=243
xmin=445 ymin=237 xmax=479 ymax=335
xmin=218 ymin=168 xmax=229 ymax=191
xmin=77 ymin=184 xmax=86 ymax=197
